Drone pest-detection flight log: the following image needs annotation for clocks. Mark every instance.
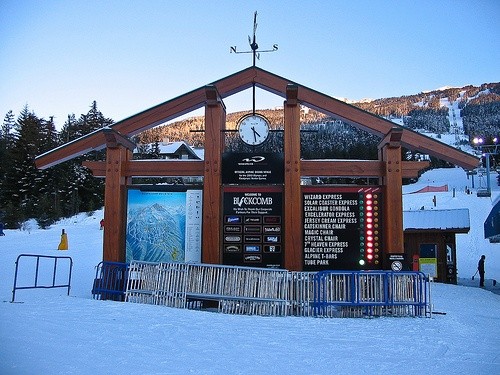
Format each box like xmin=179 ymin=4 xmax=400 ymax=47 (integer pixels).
xmin=238 ymin=116 xmax=269 ymax=146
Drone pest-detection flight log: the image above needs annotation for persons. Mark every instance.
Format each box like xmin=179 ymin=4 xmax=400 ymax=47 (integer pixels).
xmin=100 ymin=219 xmax=105 ymax=230
xmin=0 ymin=221 xmax=5 ymax=236
xmin=477 ymin=255 xmax=486 ymax=288
